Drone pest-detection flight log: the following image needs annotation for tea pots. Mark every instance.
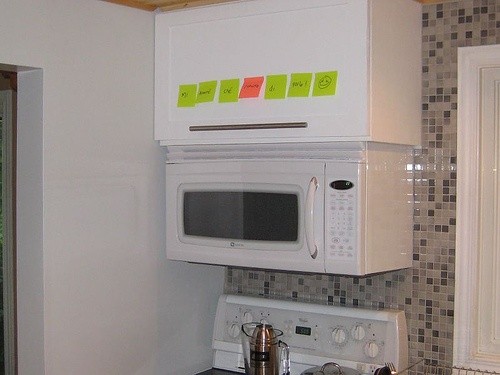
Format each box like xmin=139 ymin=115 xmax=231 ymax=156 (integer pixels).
xmin=240 ymin=321 xmax=290 ymax=375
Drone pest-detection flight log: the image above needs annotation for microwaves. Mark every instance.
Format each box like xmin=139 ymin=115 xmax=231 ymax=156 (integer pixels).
xmin=165 ymin=140 xmax=415 ymax=277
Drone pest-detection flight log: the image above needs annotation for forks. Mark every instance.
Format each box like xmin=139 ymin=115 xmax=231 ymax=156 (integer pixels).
xmin=385 ymin=362 xmax=397 ymax=375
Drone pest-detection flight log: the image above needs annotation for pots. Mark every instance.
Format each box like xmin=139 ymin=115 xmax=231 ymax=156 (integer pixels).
xmin=299 ymin=360 xmax=362 ymax=375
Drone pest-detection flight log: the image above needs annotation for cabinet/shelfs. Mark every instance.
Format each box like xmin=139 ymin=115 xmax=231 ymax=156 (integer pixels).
xmin=153 ymin=2 xmax=424 ymax=144
